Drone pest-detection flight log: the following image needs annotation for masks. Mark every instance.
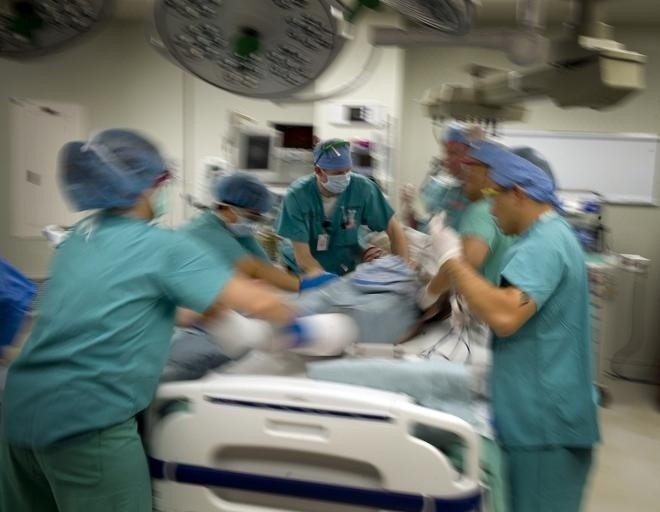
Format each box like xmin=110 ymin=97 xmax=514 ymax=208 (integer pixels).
xmin=318 ymin=172 xmax=350 ymax=194
xmin=224 ymin=212 xmax=258 ymax=239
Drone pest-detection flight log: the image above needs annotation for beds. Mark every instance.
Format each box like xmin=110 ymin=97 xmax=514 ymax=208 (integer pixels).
xmin=145 ymin=306 xmax=512 ymax=511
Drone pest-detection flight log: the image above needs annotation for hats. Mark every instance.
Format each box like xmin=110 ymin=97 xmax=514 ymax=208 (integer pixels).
xmin=314 ymin=139 xmax=351 ymax=171
xmin=445 ymin=122 xmax=554 ymax=203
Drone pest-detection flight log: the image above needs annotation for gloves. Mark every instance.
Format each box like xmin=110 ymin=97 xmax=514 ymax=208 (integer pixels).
xmin=406 ymin=229 xmax=440 ymax=274
xmin=435 ymin=228 xmax=462 ymax=263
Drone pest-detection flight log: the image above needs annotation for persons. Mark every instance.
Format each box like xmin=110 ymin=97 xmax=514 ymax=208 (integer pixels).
xmin=427 ymin=146 xmax=601 ymax=512
xmin=0 ymin=128 xmax=302 ymax=512
xmin=172 ymin=121 xmax=518 ymax=363
